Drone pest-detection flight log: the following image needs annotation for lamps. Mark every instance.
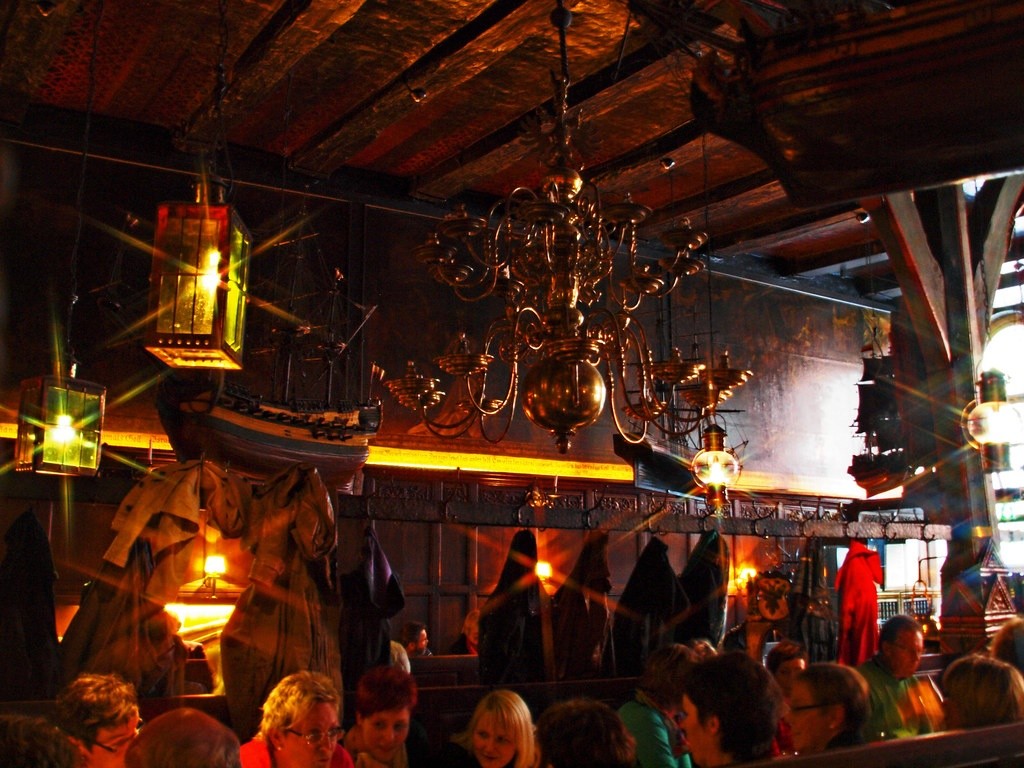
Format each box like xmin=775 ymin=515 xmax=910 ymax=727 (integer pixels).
xmin=144 ymin=0 xmax=251 ymax=370
xmin=12 ymin=0 xmax=106 ymax=478
xmin=384 ymin=0 xmax=758 ymax=508
xmin=963 ymin=178 xmax=1019 ymax=446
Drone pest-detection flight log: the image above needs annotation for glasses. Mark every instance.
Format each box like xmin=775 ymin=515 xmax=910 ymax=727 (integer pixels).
xmin=88 ymin=716 xmax=146 ymax=754
xmin=785 ymin=698 xmax=835 ymax=715
xmin=288 ymin=729 xmax=345 ymax=747
xmin=889 ymin=640 xmax=926 ymax=658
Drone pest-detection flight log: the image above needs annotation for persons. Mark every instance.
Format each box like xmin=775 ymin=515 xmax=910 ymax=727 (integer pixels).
xmin=449 ymin=610 xmax=480 ymax=655
xmin=0 ymin=713 xmax=87 ymax=768
xmin=402 ymin=621 xmax=433 ymax=656
xmin=941 ymin=654 xmax=1024 ymax=729
xmin=451 ymin=689 xmax=535 ymax=768
xmin=859 ymin=615 xmax=933 ymax=743
xmin=47 ymin=671 xmax=146 ymax=768
xmin=785 ymin=663 xmax=872 ymax=755
xmin=536 ymin=699 xmax=635 ymax=768
xmin=336 ymin=667 xmax=418 ymax=768
xmin=991 ymin=618 xmax=1024 ymax=674
xmin=766 ymin=640 xmax=806 ymax=688
xmin=124 ymin=707 xmax=241 ymax=768
xmin=616 ymin=644 xmax=705 ymax=768
xmin=679 ymin=652 xmax=784 ymax=768
xmin=240 ymin=671 xmax=355 ymax=768
